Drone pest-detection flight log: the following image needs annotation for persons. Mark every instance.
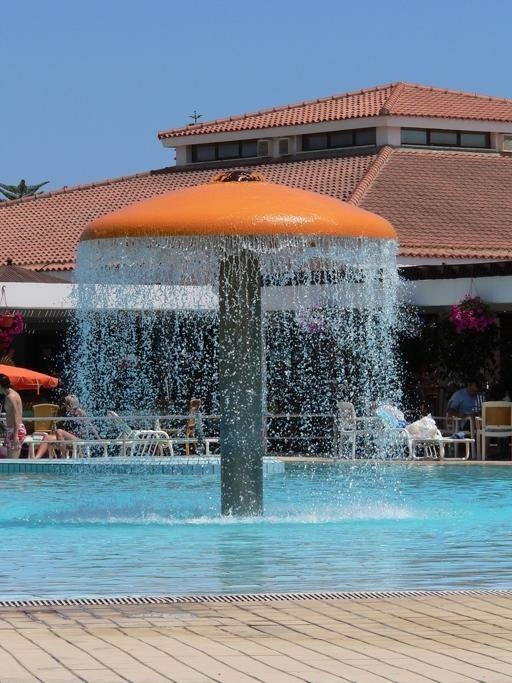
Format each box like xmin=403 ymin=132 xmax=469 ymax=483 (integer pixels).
xmin=35 ymin=395 xmax=100 ymax=458
xmin=446 ymin=377 xmax=485 ymax=431
xmin=0 ymin=374 xmax=27 ymax=459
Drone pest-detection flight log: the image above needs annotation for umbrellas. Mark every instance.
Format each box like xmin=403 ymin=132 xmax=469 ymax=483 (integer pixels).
xmin=0 ymin=364 xmax=59 ymax=390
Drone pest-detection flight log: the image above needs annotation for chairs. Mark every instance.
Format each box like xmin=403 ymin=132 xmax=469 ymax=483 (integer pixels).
xmin=332 ymin=400 xmax=510 ymax=463
xmin=1 ymin=403 xmax=175 ymax=458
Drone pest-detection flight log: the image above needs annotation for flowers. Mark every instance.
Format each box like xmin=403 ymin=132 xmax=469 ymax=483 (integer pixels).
xmin=448 ymin=264 xmax=497 ymax=335
